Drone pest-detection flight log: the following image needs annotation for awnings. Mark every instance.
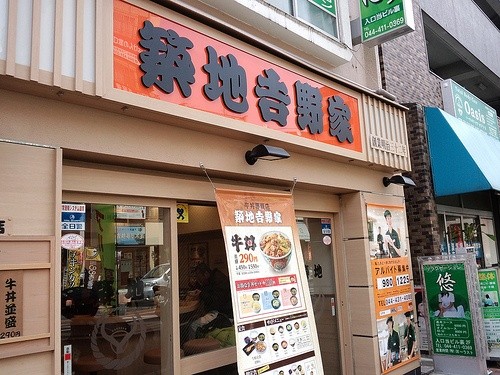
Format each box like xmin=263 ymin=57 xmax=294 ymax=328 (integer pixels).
xmin=425 ymin=107 xmax=500 ymax=197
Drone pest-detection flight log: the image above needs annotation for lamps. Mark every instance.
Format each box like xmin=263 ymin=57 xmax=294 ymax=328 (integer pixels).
xmin=383 ymin=176 xmax=416 ymax=188
xmin=245 ymin=144 xmax=290 ymax=165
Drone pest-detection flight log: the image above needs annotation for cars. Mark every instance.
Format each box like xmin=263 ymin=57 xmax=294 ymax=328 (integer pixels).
xmin=139 ymin=263 xmax=171 ymax=298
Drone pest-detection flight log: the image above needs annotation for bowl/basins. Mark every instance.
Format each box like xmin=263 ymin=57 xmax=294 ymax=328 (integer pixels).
xmin=258 ymin=231 xmax=292 ymax=272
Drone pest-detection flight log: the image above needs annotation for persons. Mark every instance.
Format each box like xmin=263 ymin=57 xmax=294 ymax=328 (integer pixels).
xmin=385 ymin=316 xmax=400 ymax=364
xmin=404 ymin=311 xmax=416 ymax=358
xmin=383 ymin=210 xmax=401 ymax=257
xmin=482 ymin=294 xmax=495 ymax=306
xmin=377 ymin=226 xmax=384 ymax=252
xmin=127 ymin=276 xmax=144 ymax=304
xmin=190 ymin=263 xmax=231 ymax=339
xmin=438 ymin=282 xmax=458 ymax=317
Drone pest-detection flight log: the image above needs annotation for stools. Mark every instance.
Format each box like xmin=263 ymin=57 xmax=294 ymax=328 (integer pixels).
xmin=183 ymin=338 xmax=220 ymax=356
xmin=72 ymin=355 xmax=112 ymax=375
xmin=144 ymin=348 xmax=184 ymax=364
